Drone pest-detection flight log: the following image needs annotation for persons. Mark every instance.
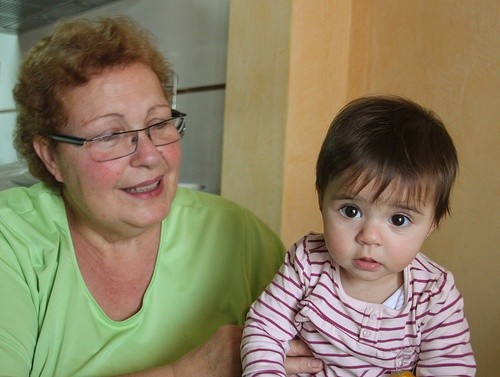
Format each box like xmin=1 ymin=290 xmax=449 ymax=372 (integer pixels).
xmin=0 ymin=15 xmax=326 ymax=377
xmin=240 ymin=93 xmax=479 ymax=376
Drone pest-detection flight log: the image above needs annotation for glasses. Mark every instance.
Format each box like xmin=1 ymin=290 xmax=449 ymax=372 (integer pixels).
xmin=48 ymin=108 xmax=187 ymax=163
